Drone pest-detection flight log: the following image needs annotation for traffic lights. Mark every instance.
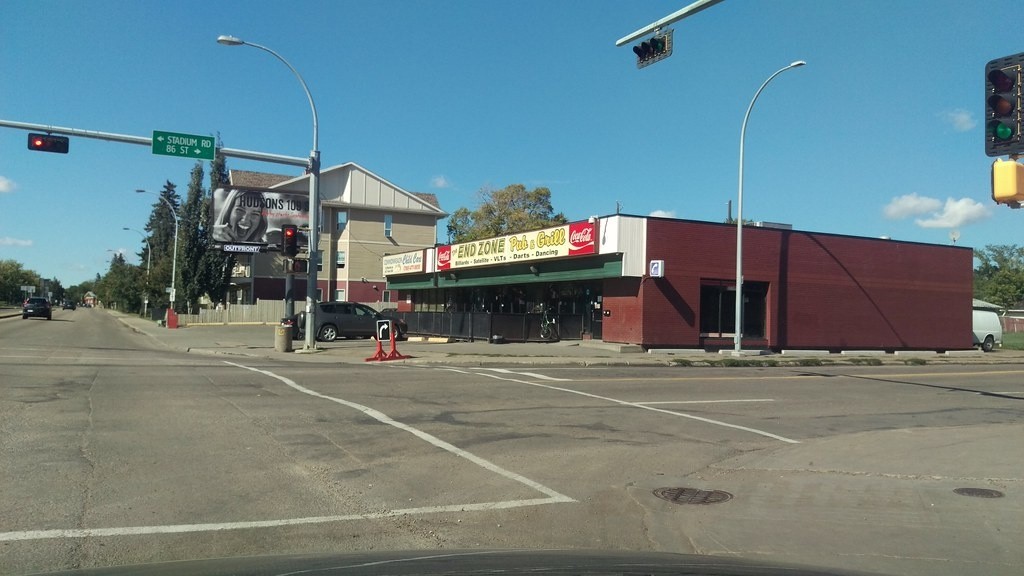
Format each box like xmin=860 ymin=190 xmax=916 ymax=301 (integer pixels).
xmin=281 ymin=224 xmax=297 ymax=256
xmin=984 ymin=52 xmax=1024 ymax=156
xmin=632 ymin=27 xmax=674 ymax=69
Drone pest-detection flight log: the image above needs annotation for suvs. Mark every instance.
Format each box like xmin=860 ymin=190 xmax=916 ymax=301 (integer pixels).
xmin=297 ymin=300 xmax=408 ymax=343
xmin=22 ymin=297 xmax=52 ymax=320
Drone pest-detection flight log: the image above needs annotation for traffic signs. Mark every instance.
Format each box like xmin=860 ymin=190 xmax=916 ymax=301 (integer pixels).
xmin=27 ymin=133 xmax=69 ymax=153
xmin=152 ymin=130 xmax=215 ymax=161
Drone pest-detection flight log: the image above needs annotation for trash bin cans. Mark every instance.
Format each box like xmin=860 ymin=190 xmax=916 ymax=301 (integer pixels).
xmin=275 ymin=325 xmax=292 ymax=352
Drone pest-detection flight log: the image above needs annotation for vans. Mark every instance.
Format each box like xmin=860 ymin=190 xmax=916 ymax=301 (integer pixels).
xmin=972 ymin=309 xmax=1002 ymax=352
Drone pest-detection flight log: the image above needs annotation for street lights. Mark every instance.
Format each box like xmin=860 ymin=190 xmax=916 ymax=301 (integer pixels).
xmin=136 ymin=189 xmax=179 ymax=310
xmin=734 ymin=60 xmax=807 ymax=352
xmin=123 ymin=228 xmax=151 ymax=318
xmin=216 ymin=33 xmax=323 ymax=353
xmin=106 ymin=249 xmax=130 ymax=278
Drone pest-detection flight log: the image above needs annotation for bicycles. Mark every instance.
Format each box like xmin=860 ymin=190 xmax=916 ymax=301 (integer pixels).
xmin=540 ymin=310 xmax=555 ymax=340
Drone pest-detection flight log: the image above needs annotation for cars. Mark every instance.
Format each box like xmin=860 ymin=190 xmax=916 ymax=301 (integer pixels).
xmin=280 ymin=313 xmax=305 ymax=339
xmin=77 ymin=301 xmax=92 ymax=308
xmin=63 ymin=300 xmax=75 ymax=311
